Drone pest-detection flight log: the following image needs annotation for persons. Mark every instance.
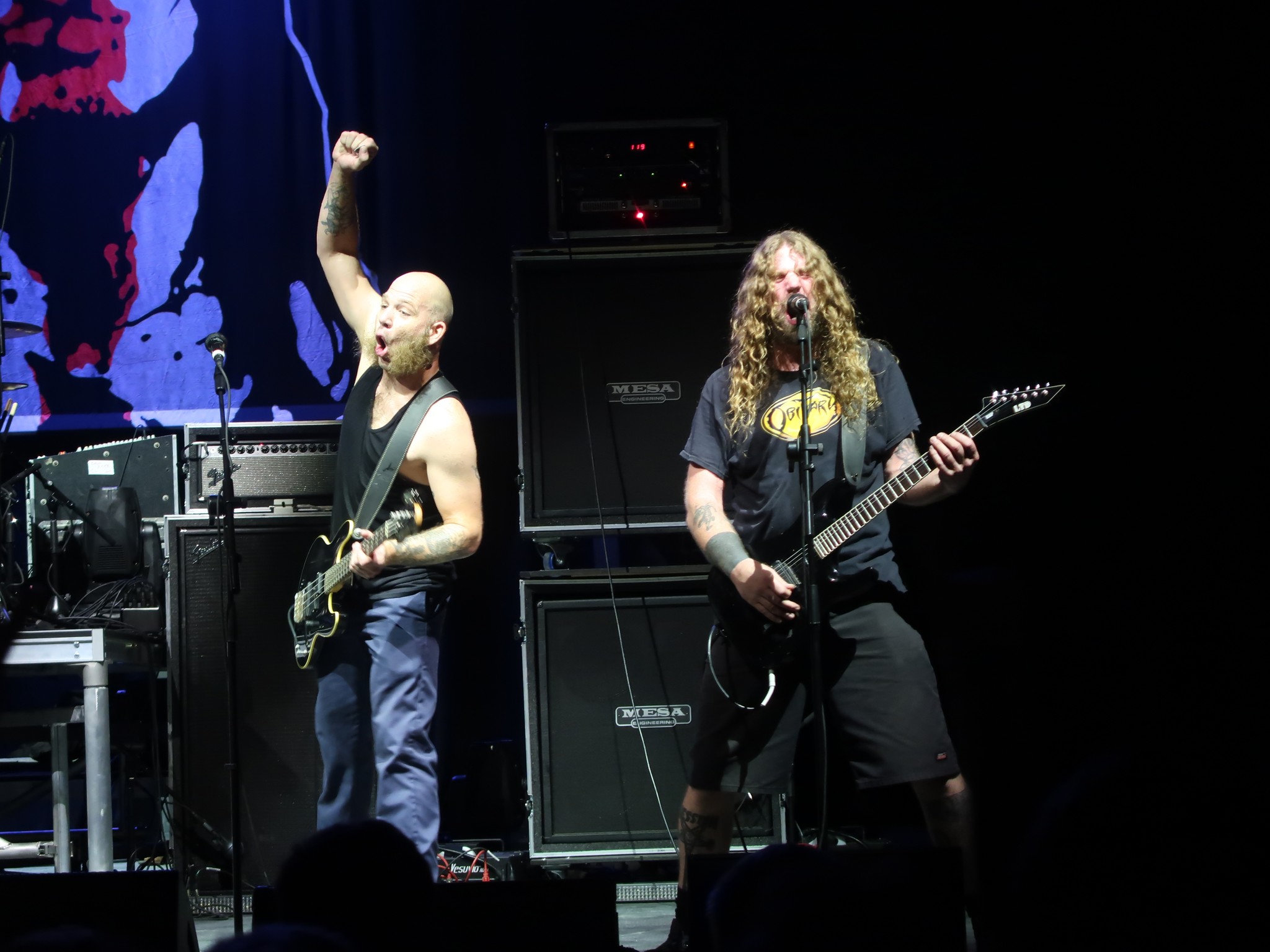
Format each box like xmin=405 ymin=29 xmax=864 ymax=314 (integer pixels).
xmin=315 ymin=129 xmax=484 ymax=886
xmin=648 ymin=229 xmax=974 ymax=952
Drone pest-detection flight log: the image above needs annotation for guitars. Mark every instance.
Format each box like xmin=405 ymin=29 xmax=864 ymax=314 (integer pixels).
xmin=704 ymin=378 xmax=1070 ymax=716
xmin=288 ymin=485 xmax=428 ymax=673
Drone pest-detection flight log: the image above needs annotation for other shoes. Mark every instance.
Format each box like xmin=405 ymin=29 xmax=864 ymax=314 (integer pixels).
xmin=644 ymin=919 xmax=690 ymax=952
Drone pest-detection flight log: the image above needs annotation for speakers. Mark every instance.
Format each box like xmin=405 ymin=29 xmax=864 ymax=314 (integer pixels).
xmin=535 ymin=593 xmax=789 ymax=845
xmin=163 ymin=511 xmax=334 ymax=898
xmin=0 ymin=870 xmax=201 ymax=952
xmin=667 ymin=848 xmax=969 ymax=951
xmin=524 ymin=245 xmax=763 ymax=522
xmin=251 ymin=879 xmax=621 ymax=952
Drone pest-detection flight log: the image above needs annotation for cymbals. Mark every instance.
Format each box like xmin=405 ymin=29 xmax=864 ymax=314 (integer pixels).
xmin=2 ymin=318 xmax=43 ymax=340
xmin=2 ymin=382 xmax=28 ymax=392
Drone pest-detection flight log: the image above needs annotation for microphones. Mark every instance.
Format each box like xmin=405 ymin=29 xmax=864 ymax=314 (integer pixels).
xmin=204 ymin=332 xmax=228 ymax=366
xmin=13 ymin=455 xmax=34 ymax=471
xmin=786 ymin=293 xmax=810 ymax=317
